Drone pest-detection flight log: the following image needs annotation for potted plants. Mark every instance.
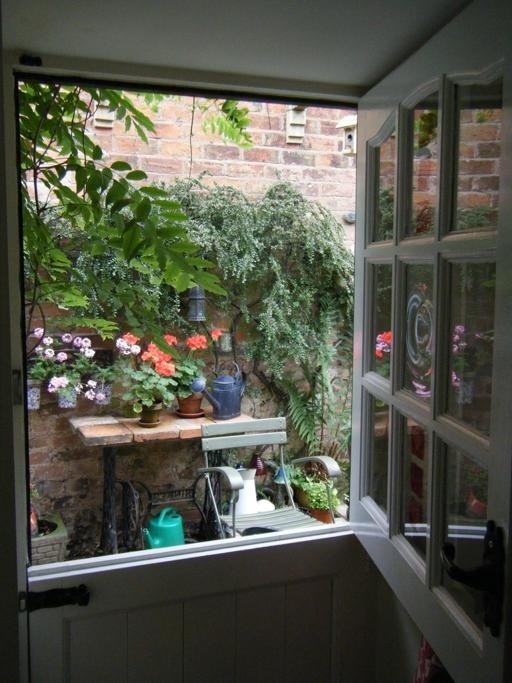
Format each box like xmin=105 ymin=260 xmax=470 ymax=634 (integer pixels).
xmin=30 ymin=473 xmax=68 ymax=566
xmin=302 ymin=480 xmax=340 ymax=523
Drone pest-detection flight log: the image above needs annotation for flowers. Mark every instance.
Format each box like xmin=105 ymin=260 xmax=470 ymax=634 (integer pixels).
xmin=373 ymin=284 xmax=465 ymax=393
xmin=26 ymin=327 xmax=222 ymax=427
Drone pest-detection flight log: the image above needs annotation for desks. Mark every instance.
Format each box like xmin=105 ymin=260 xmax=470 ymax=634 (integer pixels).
xmin=68 ymin=406 xmax=254 ymax=553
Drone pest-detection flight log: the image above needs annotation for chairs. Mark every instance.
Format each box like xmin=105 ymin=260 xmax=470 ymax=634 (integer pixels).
xmin=198 ymin=417 xmax=342 ymax=538
